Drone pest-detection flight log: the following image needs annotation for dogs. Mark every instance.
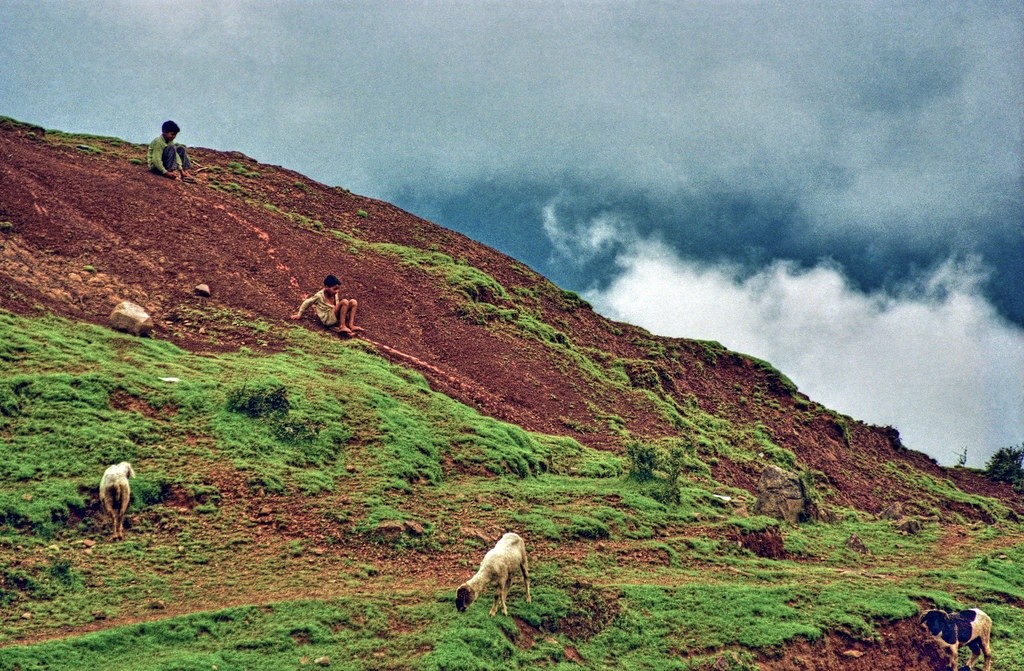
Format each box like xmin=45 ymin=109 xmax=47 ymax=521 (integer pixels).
xmin=918 ymin=608 xmax=992 ymax=671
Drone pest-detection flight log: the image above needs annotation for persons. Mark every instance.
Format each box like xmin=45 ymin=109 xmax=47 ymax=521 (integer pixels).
xmin=289 ymin=275 xmax=365 ymax=334
xmin=147 ymin=120 xmax=198 ymax=184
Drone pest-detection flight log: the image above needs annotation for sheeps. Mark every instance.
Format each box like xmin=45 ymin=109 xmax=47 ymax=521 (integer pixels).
xmin=456 ymin=532 xmax=532 ymax=618
xmin=99 ymin=461 xmax=136 ymax=540
